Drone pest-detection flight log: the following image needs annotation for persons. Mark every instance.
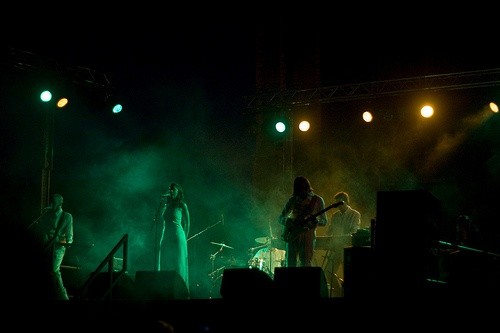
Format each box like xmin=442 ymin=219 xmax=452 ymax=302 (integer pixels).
xmin=280 ymin=176 xmax=327 ymax=266
xmin=158 ymin=182 xmax=190 ymax=290
xmin=41 ymin=195 xmax=73 ymax=300
xmin=252 ymin=246 xmax=284 ymax=274
xmin=326 ymin=192 xmax=361 ymax=297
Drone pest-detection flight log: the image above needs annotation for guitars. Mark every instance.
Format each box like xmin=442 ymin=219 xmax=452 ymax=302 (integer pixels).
xmin=284 ymin=200 xmax=346 ymax=242
xmin=33 ymin=233 xmax=95 ymax=255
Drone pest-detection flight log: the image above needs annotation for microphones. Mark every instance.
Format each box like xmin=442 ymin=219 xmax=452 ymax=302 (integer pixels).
xmin=43 ymin=207 xmax=52 ymax=210
xmin=162 ymin=194 xmax=171 ymax=197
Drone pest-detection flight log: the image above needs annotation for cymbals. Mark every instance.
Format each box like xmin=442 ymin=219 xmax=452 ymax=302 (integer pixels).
xmin=210 ymin=242 xmax=235 ymax=249
xmin=255 ymin=237 xmax=273 ymax=245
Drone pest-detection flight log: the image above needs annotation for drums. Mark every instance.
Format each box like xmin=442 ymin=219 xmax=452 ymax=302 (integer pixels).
xmin=250 ymin=259 xmax=268 ymax=273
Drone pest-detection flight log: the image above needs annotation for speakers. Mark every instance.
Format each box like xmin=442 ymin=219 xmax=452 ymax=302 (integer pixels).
xmin=218 ymin=268 xmax=276 ymax=299
xmin=272 ymin=266 xmax=330 ymax=298
xmin=135 ymin=271 xmax=189 ymax=300
xmin=86 ymin=271 xmax=138 ymax=302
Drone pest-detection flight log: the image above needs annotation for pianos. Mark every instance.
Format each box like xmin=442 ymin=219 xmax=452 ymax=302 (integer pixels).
xmin=271 ymin=235 xmax=352 ymax=299
